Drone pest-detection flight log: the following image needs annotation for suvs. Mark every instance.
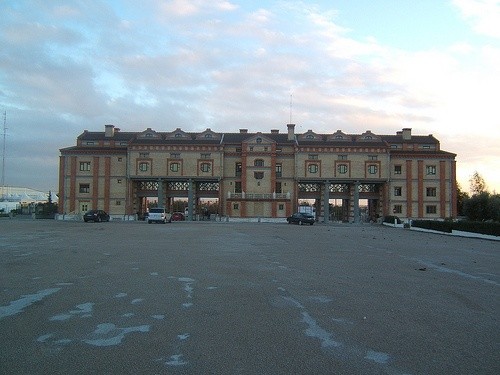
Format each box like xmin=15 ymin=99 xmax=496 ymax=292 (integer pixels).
xmin=148 ymin=208 xmax=171 ymax=223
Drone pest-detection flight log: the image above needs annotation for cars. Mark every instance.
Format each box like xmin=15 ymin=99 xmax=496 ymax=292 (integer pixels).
xmin=171 ymin=212 xmax=185 ymax=223
xmin=286 ymin=212 xmax=314 ymax=226
xmin=82 ymin=209 xmax=111 ymax=223
xmin=143 ymin=211 xmax=148 ymax=220
